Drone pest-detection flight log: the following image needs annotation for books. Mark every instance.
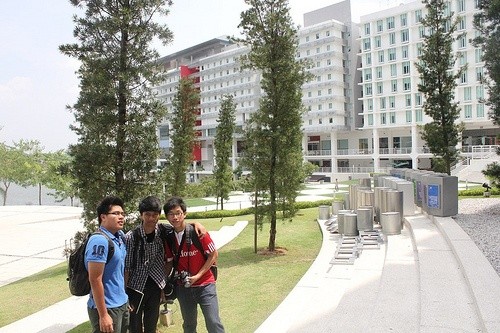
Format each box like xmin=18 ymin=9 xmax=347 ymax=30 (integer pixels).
xmin=124 ymin=285 xmax=144 ymax=314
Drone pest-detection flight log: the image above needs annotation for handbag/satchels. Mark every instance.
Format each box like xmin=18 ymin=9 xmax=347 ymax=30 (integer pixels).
xmin=164 ymin=273 xmax=178 ymax=300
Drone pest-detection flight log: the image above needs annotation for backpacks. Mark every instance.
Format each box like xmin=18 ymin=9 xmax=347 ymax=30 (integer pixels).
xmin=66 ymin=230 xmax=115 ymax=297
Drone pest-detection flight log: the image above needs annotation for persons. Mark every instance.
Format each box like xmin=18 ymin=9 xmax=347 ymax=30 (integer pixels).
xmin=84 ymin=195 xmax=133 ymax=333
xmin=163 ymin=198 xmax=227 ymax=333
xmin=121 ymin=195 xmax=208 ymax=333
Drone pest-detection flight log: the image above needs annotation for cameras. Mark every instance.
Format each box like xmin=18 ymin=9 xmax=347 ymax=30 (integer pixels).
xmin=177 ymin=270 xmax=191 ymax=287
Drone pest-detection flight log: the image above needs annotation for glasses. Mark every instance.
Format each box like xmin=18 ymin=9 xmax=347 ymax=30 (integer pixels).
xmin=106 ymin=211 xmax=126 ymax=217
xmin=167 ymin=212 xmax=185 ymax=217
